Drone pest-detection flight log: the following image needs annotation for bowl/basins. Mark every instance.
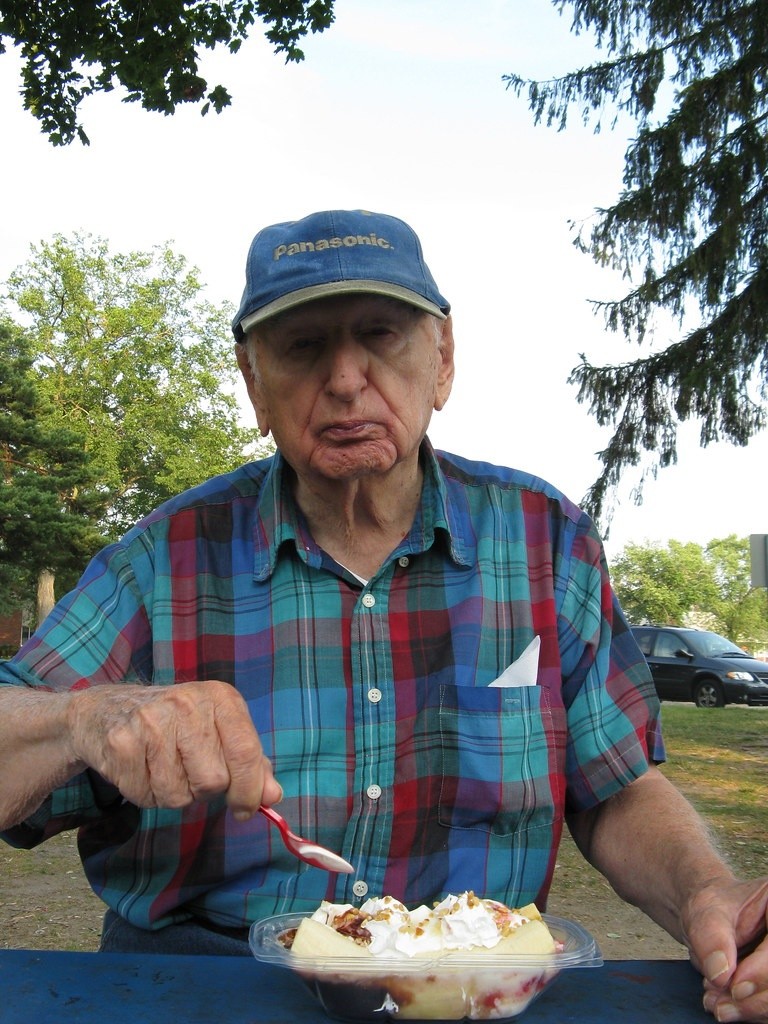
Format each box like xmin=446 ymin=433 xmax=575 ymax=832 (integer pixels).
xmin=248 ymin=911 xmax=604 ymax=1024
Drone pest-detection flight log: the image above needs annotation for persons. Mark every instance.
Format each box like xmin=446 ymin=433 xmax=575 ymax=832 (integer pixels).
xmin=0 ymin=211 xmax=768 ymax=1024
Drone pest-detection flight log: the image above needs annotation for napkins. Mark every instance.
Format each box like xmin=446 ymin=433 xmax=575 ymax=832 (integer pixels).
xmin=485 ymin=634 xmax=542 ymax=688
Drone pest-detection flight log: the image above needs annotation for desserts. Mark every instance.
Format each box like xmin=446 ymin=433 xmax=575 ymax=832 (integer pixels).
xmin=279 ymin=889 xmax=564 ymax=1019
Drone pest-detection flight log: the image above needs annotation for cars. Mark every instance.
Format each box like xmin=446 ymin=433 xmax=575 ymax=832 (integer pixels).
xmin=629 ymin=623 xmax=768 ymax=708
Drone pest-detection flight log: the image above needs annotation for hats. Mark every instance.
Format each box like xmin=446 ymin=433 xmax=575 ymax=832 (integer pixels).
xmin=232 ymin=208 xmax=451 ymax=343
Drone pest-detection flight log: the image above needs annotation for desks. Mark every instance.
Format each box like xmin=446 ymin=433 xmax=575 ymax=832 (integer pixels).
xmin=0 ymin=950 xmax=717 ymax=1024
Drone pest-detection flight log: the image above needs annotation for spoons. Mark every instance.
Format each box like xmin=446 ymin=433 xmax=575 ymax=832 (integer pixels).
xmin=258 ymin=805 xmax=356 ymax=873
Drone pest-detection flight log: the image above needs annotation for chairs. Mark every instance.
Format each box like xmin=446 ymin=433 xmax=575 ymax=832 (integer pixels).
xmin=658 ymin=638 xmax=675 ymax=658
xmin=639 ymin=636 xmax=650 ymax=655
